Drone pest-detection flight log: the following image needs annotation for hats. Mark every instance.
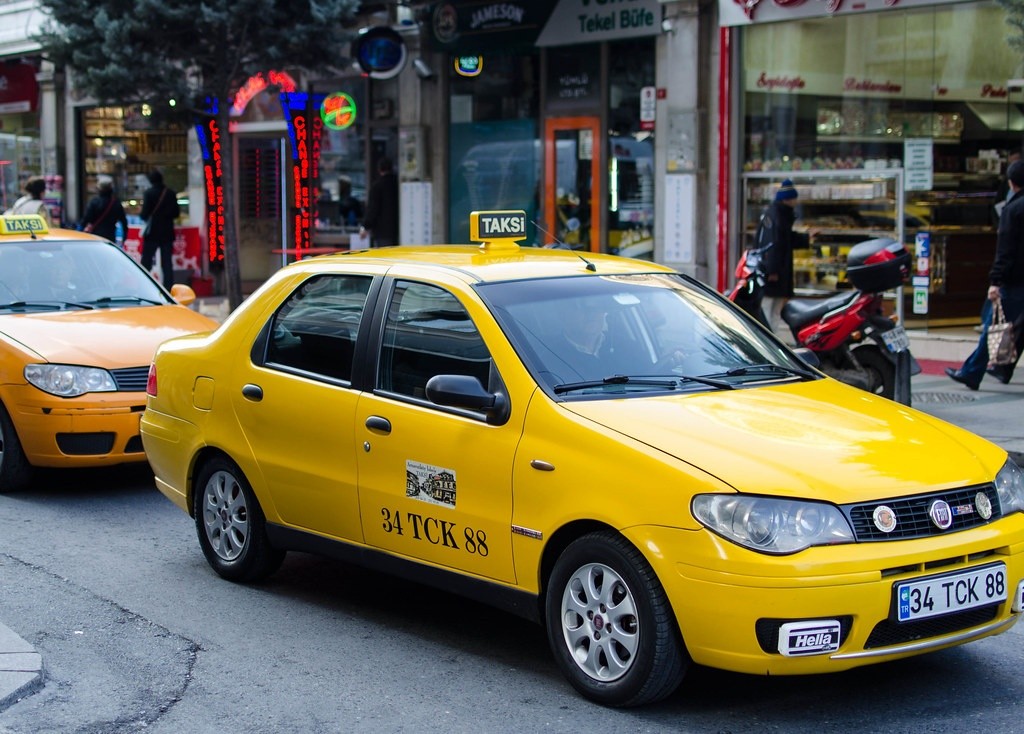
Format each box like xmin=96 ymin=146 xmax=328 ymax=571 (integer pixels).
xmin=97 ymin=176 xmax=113 ymax=191
xmin=776 ymin=178 xmax=798 ymax=200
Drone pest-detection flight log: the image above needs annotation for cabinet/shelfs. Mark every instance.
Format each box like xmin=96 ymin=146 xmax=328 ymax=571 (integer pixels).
xmin=741 ymin=156 xmax=907 ymax=327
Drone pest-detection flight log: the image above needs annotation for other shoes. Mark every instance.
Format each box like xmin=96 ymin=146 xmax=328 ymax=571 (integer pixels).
xmin=985 ymin=367 xmax=1011 ymax=384
xmin=944 ymin=367 xmax=980 ymax=390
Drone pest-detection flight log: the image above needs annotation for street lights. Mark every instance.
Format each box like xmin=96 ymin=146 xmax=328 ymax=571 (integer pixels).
xmin=1005 ymin=86 xmax=1021 ymax=166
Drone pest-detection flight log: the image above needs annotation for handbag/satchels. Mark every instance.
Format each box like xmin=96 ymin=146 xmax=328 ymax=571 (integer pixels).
xmin=987 ymin=297 xmax=1017 ymax=365
xmin=85 ymin=226 xmax=93 ymax=233
xmin=140 ymin=216 xmax=153 ymax=238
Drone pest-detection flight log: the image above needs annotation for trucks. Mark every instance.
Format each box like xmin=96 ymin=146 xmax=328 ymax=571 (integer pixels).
xmin=458 ymin=139 xmax=655 ymax=222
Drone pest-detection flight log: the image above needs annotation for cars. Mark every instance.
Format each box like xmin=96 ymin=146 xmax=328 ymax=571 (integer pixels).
xmin=450 ymin=197 xmax=653 ymax=258
xmin=0 ymin=214 xmax=221 ymax=492
xmin=140 ymin=209 xmax=1024 ymax=710
xmin=313 ymin=165 xmax=378 ymax=245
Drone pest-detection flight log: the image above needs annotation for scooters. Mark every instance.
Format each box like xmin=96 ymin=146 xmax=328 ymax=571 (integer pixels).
xmin=724 ymin=213 xmax=922 ymax=400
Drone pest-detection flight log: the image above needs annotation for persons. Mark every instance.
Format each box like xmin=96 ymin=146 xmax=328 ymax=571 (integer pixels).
xmin=137 ymin=167 xmax=179 ymax=295
xmin=943 ymin=147 xmax=1024 ymax=391
xmin=357 ymin=154 xmax=399 ymax=244
xmin=3 ymin=179 xmax=54 ymax=230
xmin=77 ymin=176 xmax=130 ymax=246
xmin=754 ymin=178 xmax=816 ymax=295
